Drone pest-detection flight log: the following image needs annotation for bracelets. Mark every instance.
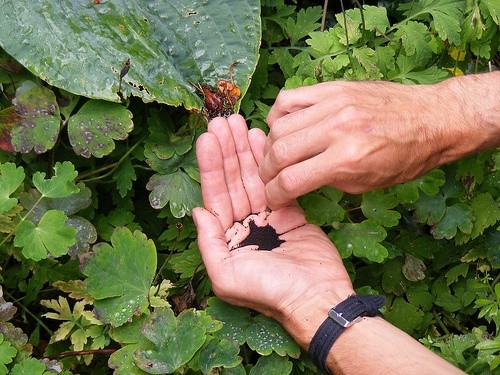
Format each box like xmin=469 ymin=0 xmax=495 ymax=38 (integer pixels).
xmin=307 ymin=294 xmax=386 ymax=373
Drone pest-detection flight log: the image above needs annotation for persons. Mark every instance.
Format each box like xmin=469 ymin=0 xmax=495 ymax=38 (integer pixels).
xmin=193 ymin=66 xmax=499 ymax=374
